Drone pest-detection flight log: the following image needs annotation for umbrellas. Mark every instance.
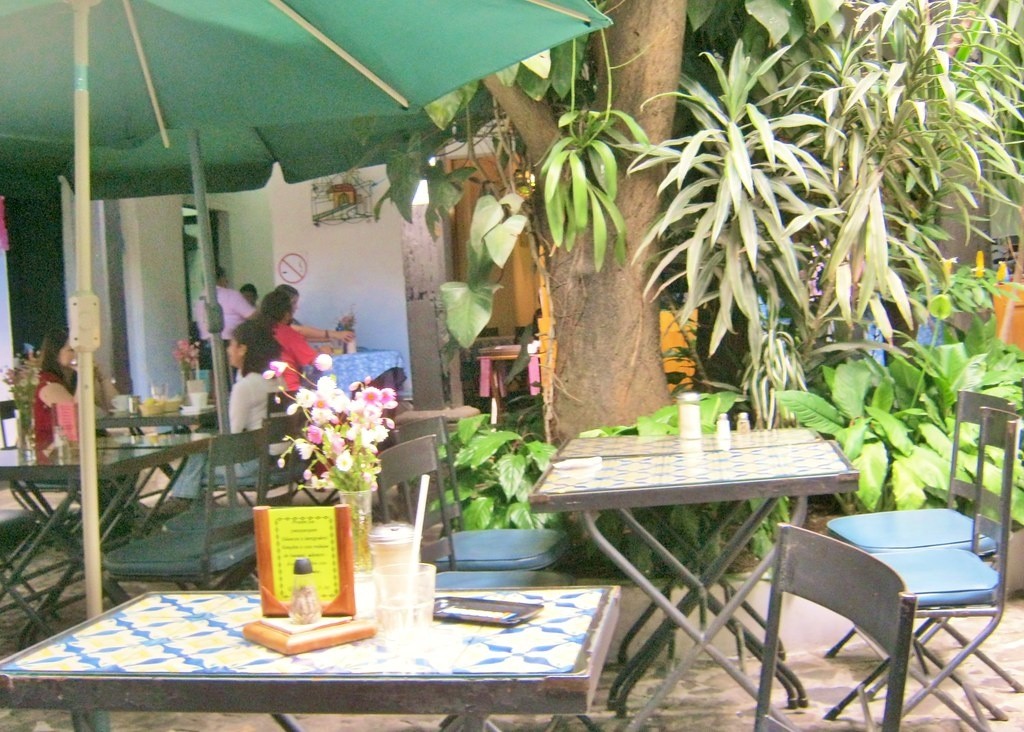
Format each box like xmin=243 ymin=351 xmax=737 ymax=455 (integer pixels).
xmin=0 ymin=0 xmax=613 ymax=731
xmin=0 ymin=111 xmax=467 ymax=510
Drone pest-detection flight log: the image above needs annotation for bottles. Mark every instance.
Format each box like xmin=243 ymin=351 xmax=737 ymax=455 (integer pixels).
xmin=717 ymin=413 xmax=729 ymax=434
xmin=679 ymin=391 xmax=701 ymax=439
xmin=737 ymin=412 xmax=750 ymax=434
xmin=368 ymin=523 xmax=423 ymax=565
xmin=129 ymin=396 xmax=141 ymax=413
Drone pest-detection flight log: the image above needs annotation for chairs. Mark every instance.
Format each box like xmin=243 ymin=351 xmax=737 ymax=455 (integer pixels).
xmin=753 ymin=523 xmax=917 ymax=732
xmin=105 ymin=389 xmax=610 ymax=732
xmin=827 ymin=389 xmax=1024 ymax=701
xmin=824 ymin=405 xmax=1008 ymax=732
xmin=1 ymin=399 xmax=83 ymax=547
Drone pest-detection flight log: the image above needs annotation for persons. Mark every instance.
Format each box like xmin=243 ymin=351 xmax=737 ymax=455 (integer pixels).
xmin=156 ymin=322 xmax=288 ymax=515
xmin=260 ymin=290 xmax=332 ymax=390
xmin=196 ymin=266 xmax=259 ymax=369
xmin=276 ymin=284 xmax=355 ymax=344
xmin=240 ymin=283 xmax=257 ymax=305
xmin=33 ymin=326 xmax=139 ymax=500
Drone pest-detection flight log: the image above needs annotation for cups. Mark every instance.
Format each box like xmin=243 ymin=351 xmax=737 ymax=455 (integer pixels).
xmin=190 ymin=392 xmax=207 ymax=405
xmin=151 ymin=383 xmax=169 ymax=399
xmin=112 ymin=395 xmax=129 ymax=408
xmin=372 ymin=562 xmax=437 ymax=651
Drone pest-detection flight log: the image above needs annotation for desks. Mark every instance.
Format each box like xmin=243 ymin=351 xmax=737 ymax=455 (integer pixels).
xmin=0 ymin=405 xmax=218 ymax=653
xmin=529 ymin=426 xmax=859 ymax=719
xmin=0 ymin=585 xmax=621 ymax=732
xmin=300 ymin=347 xmax=404 ymax=401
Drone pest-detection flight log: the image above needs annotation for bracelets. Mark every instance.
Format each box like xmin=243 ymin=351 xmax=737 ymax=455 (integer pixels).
xmin=325 ymin=330 xmax=328 ymax=337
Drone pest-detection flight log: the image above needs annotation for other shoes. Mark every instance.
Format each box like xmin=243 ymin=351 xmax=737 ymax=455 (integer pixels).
xmin=152 ymin=500 xmax=191 ymax=519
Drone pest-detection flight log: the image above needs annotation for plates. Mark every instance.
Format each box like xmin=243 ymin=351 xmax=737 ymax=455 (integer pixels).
xmin=108 ymin=408 xmax=128 ymax=412
xmin=181 ymin=405 xmax=214 ymax=410
